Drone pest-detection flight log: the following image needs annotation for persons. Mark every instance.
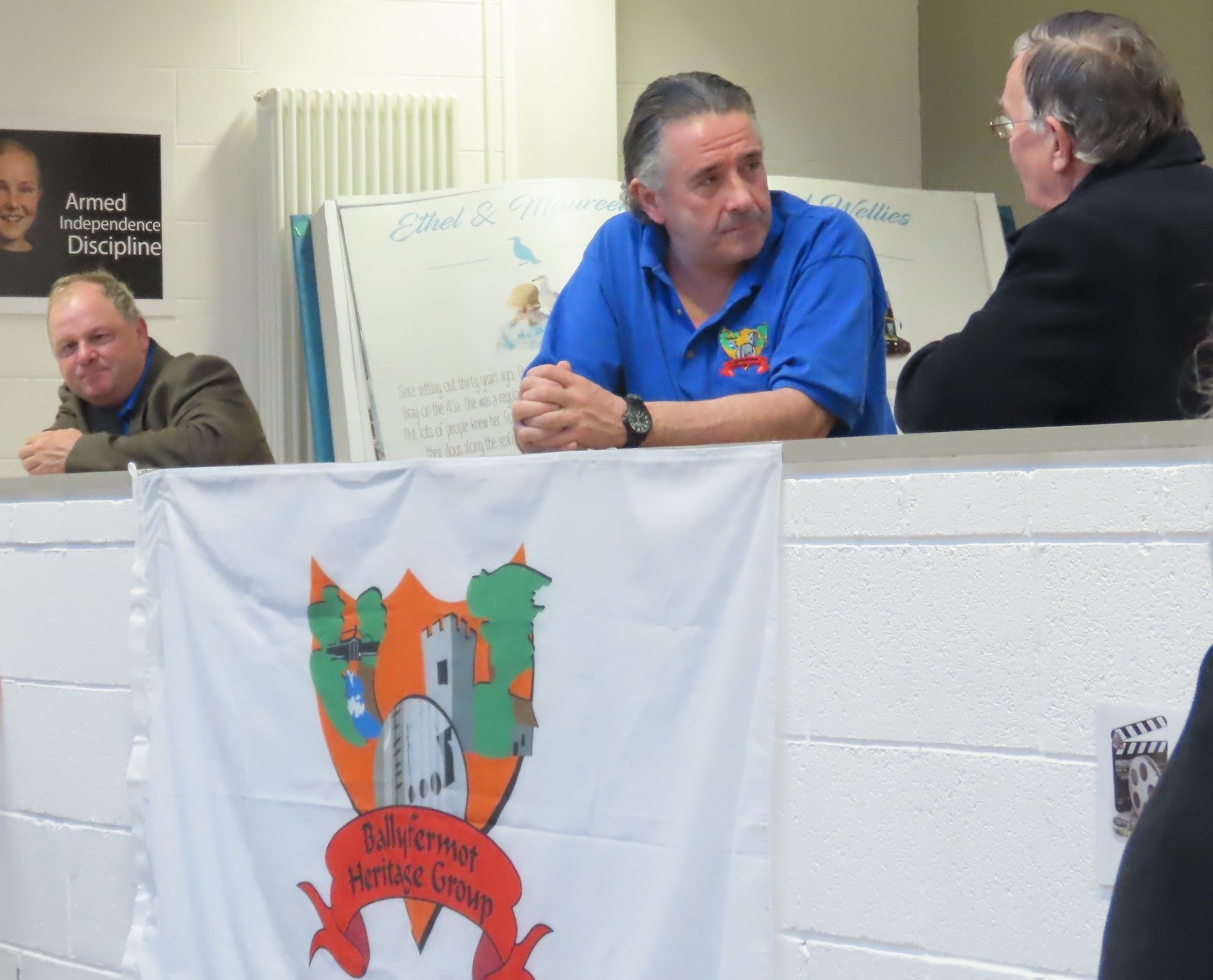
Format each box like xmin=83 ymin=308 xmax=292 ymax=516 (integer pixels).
xmin=895 ymin=9 xmax=1213 ymax=434
xmin=513 ymin=71 xmax=898 ymax=453
xmin=0 ymin=138 xmax=52 ymax=296
xmin=19 ymin=269 xmax=275 ymax=475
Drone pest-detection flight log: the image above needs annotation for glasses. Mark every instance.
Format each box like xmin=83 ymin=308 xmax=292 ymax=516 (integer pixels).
xmin=988 ymin=115 xmax=1039 ymax=140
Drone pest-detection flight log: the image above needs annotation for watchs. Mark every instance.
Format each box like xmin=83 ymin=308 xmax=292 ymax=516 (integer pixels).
xmin=616 ymin=393 xmax=652 ymax=449
